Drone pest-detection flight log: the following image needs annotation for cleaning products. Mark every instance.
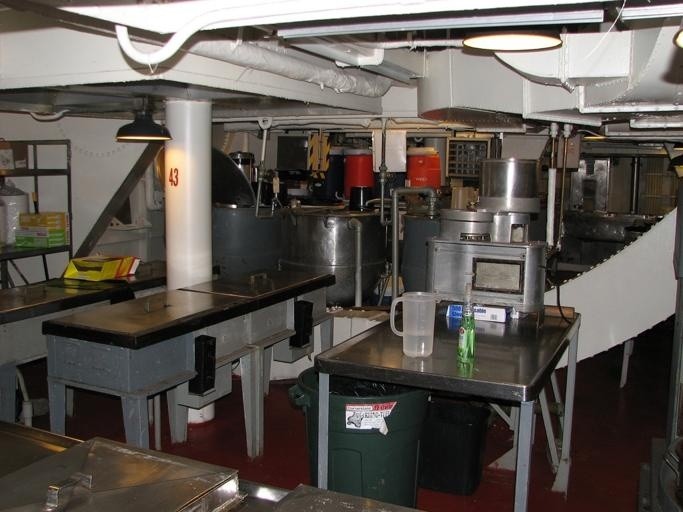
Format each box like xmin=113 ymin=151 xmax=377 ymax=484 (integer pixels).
xmin=456 ymin=363 xmax=473 ymax=379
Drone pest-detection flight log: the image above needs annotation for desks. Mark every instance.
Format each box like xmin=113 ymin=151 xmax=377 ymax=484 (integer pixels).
xmin=43 ymin=269 xmax=335 ymax=457
xmin=2 ymin=256 xmax=220 ymax=425
xmin=312 ymin=301 xmax=582 ymax=511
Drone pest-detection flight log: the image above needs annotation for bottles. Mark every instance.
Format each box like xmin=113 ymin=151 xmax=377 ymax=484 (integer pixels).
xmin=348 ymin=186 xmax=373 ymax=211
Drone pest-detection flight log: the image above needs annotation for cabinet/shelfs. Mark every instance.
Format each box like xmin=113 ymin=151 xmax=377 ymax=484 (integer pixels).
xmin=0 ymin=140 xmax=75 ymax=260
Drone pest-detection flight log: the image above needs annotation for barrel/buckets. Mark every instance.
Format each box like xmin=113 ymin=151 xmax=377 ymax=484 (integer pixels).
xmin=289 ymin=366 xmax=425 ymax=509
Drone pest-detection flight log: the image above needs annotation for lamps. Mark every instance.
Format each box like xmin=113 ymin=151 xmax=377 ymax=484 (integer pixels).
xmin=671 ymin=17 xmax=683 ymax=50
xmin=272 ymin=3 xmax=607 ymax=40
xmin=111 ymin=97 xmax=176 ymax=144
xmin=615 ymin=3 xmax=683 ymax=22
xmin=461 ymin=24 xmax=564 ymax=55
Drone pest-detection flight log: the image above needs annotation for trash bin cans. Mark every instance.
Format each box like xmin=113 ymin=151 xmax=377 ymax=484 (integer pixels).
xmin=287 ymin=366 xmax=492 ymax=509
